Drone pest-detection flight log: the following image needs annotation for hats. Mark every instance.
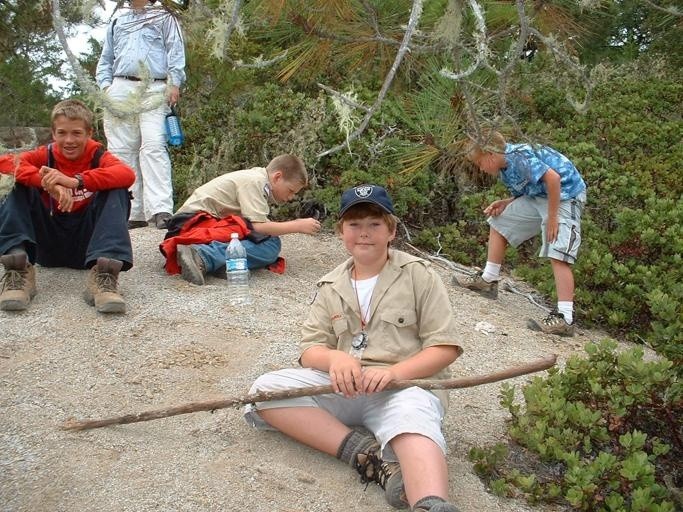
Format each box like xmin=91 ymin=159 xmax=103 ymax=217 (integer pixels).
xmin=338 ymin=185 xmax=394 ymax=219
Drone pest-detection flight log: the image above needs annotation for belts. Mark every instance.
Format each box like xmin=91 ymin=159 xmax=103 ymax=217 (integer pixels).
xmin=121 ymin=76 xmax=167 ymax=82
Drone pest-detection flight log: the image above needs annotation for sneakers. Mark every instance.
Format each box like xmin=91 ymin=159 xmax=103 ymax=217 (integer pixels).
xmin=528 ymin=309 xmax=575 ymax=336
xmin=83 ymin=257 xmax=126 ymax=312
xmin=214 ymin=264 xmax=250 ymax=281
xmin=0 ymin=253 xmax=36 ymax=310
xmin=155 ymin=212 xmax=172 ymax=229
xmin=357 ymin=444 xmax=410 ymax=509
xmin=453 ymin=270 xmax=498 ymax=298
xmin=176 ymin=244 xmax=206 ymax=285
xmin=128 ymin=220 xmax=147 ymax=229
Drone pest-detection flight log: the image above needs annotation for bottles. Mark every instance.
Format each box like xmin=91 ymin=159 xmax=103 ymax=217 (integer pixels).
xmin=225 ymin=232 xmax=251 ymax=307
xmin=165 ymin=106 xmax=183 ymax=147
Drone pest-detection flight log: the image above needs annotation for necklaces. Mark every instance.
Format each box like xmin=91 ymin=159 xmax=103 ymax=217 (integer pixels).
xmin=351 ymin=266 xmax=386 ymax=349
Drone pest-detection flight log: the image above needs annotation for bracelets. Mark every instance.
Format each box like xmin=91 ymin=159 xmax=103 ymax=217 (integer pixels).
xmin=73 ymin=173 xmax=83 ymax=190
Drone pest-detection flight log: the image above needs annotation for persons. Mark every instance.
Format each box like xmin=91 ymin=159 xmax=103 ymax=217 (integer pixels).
xmin=0 ymin=100 xmax=135 ymax=314
xmin=245 ymin=185 xmax=464 ymax=512
xmin=450 ymin=128 xmax=587 ymax=338
xmin=158 ymin=153 xmax=321 ymax=286
xmin=96 ymin=0 xmax=186 ymax=230
